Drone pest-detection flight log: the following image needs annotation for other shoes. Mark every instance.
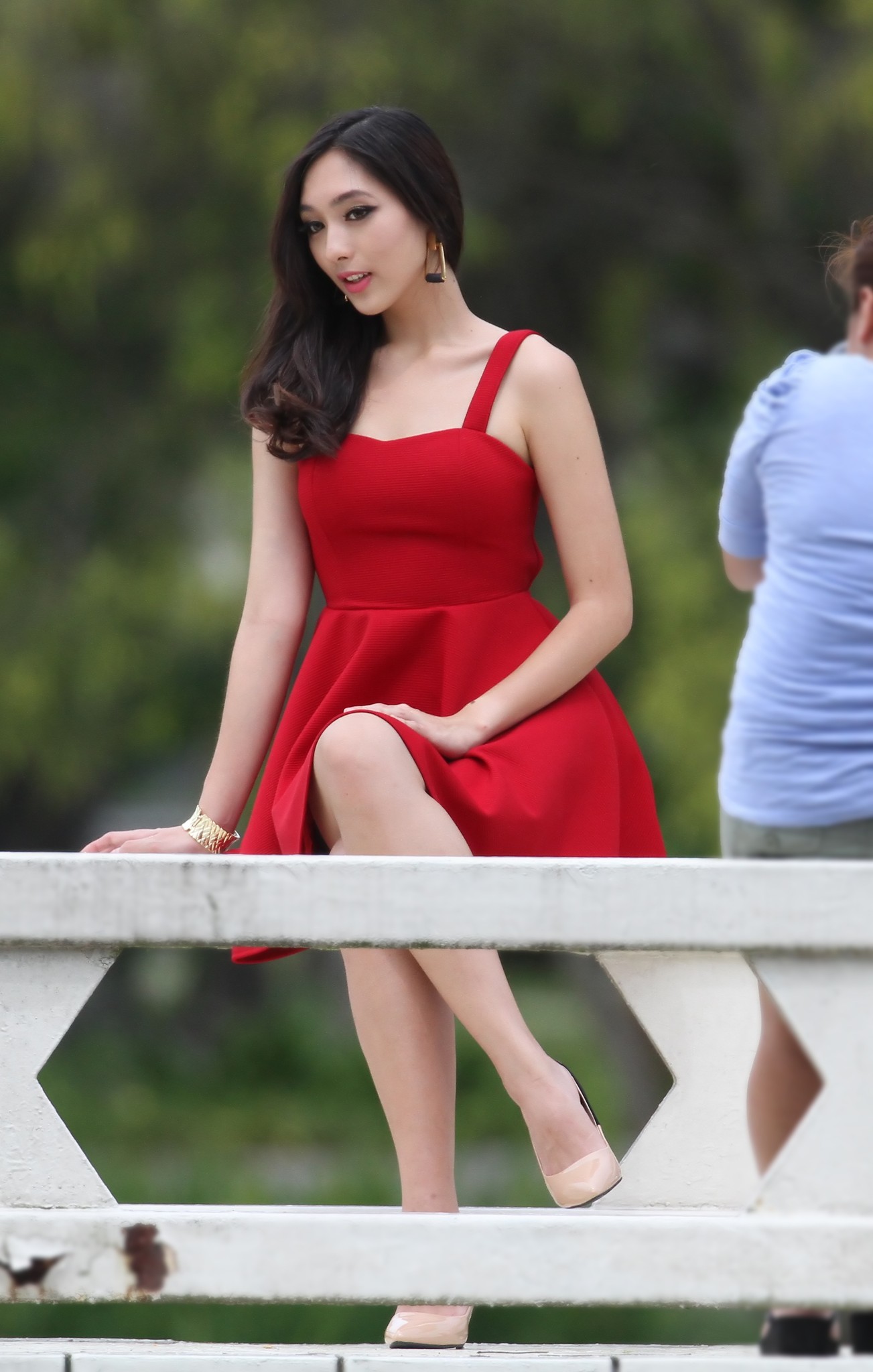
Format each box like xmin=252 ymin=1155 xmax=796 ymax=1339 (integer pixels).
xmin=849 ymin=1306 xmax=873 ymax=1356
xmin=757 ymin=1303 xmax=842 ymax=1355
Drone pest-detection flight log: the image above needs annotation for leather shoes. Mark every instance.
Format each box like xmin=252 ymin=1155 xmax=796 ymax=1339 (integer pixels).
xmin=381 ymin=1300 xmax=474 ymax=1351
xmin=518 ymin=1061 xmax=622 ymax=1209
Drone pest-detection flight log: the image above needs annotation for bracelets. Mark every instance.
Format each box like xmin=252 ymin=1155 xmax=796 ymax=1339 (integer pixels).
xmin=183 ymin=804 xmax=240 ymax=854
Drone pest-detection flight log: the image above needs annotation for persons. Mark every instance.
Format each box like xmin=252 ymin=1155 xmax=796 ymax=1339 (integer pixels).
xmin=82 ymin=110 xmax=666 ymax=1344
xmin=718 ymin=225 xmax=873 ymax=1353
